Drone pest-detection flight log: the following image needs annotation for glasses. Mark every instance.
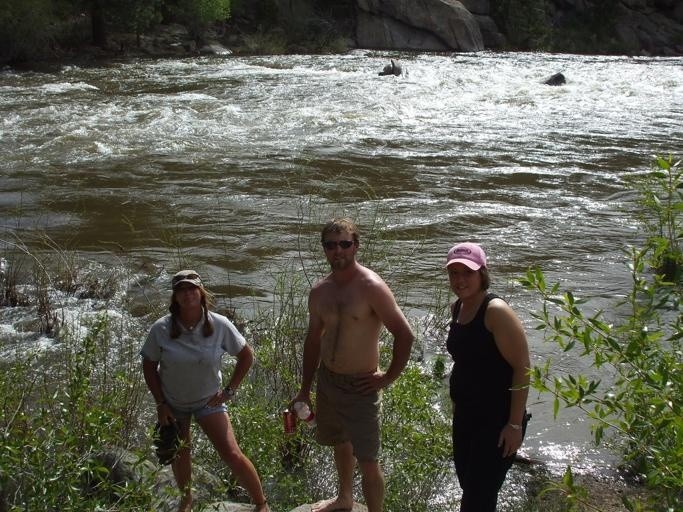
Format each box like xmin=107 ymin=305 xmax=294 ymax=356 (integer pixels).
xmin=174 ymin=274 xmax=203 ymax=284
xmin=323 ymin=240 xmax=355 ymax=249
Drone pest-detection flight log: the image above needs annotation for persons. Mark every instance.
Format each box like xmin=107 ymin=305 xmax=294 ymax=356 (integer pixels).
xmin=287 ymin=217 xmax=415 ymax=512
xmin=138 ymin=269 xmax=274 ymax=512
xmin=444 ymin=241 xmax=532 ymax=511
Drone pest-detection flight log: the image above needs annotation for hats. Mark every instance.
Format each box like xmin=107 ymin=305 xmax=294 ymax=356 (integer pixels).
xmin=445 ymin=243 xmax=486 ymax=271
xmin=171 ymin=270 xmax=204 ymax=289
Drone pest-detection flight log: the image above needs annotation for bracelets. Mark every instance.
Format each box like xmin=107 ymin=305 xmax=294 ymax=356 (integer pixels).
xmin=155 ymin=400 xmax=165 ymax=406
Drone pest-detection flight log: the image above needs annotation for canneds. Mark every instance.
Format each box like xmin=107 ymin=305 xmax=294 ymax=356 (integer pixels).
xmin=283 ymin=409 xmax=296 ymax=434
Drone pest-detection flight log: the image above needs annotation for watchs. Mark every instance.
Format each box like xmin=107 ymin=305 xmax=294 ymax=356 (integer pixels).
xmin=508 ymin=422 xmax=523 ymax=433
xmin=225 ymin=385 xmax=234 ymax=398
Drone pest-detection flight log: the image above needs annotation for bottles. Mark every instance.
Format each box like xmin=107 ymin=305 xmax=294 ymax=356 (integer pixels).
xmin=294 ymin=401 xmax=317 ymax=428
